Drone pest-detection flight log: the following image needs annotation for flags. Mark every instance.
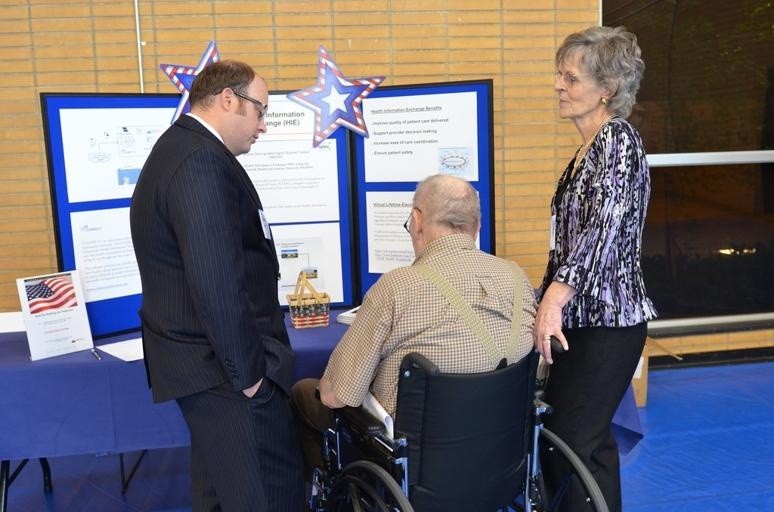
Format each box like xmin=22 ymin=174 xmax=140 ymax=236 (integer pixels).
xmin=22 ymin=272 xmax=80 ymax=314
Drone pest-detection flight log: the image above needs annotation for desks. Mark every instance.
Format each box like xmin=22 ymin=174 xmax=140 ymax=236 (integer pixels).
xmin=0 ymin=308 xmax=345 ymax=507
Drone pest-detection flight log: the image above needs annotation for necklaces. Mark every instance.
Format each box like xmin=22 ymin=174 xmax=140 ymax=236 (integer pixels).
xmin=573 ymin=116 xmax=614 ymax=167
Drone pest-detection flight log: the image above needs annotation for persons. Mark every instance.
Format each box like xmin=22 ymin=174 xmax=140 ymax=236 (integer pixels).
xmin=529 ymin=26 xmax=653 ymax=511
xmin=128 ymin=60 xmax=308 ymax=512
xmin=285 ymin=173 xmax=537 ymax=446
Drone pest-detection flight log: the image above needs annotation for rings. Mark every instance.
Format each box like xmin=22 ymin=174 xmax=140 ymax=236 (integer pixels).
xmin=543 ymin=335 xmax=551 ymax=341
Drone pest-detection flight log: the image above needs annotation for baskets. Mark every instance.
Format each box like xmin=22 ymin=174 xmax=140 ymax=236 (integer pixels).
xmin=285 ymin=271 xmax=330 ymax=330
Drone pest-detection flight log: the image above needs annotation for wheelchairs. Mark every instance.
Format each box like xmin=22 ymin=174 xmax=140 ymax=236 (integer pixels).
xmin=305 ymin=326 xmax=612 ymax=512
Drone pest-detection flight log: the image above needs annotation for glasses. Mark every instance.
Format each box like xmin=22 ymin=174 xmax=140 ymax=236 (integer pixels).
xmin=213 ymin=88 xmax=269 ymax=118
xmin=554 ymin=69 xmax=602 ymax=84
xmin=403 ymin=206 xmax=423 ymax=233
